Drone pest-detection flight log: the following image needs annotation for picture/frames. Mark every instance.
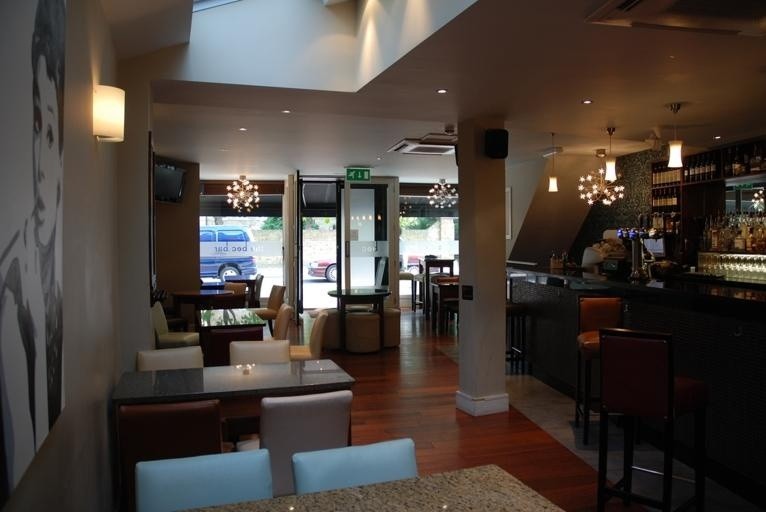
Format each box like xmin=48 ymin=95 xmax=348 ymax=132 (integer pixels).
xmin=505 ymin=186 xmax=512 ymax=239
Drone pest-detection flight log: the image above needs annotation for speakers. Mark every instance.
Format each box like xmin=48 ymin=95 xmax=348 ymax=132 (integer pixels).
xmin=484 ymin=127 xmax=508 ymax=159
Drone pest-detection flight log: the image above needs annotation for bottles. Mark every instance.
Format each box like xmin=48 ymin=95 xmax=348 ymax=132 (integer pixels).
xmin=652 ymin=212 xmax=678 ymax=233
xmin=549 ymin=250 xmax=569 ymax=270
xmin=700 ymin=210 xmax=766 ymax=253
xmin=726 ymin=147 xmax=766 ymax=174
xmin=652 ymin=156 xmax=718 ymax=213
xmin=703 ymin=255 xmax=766 ymax=278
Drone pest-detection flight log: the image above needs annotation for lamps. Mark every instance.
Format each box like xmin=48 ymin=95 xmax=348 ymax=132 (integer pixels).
xmin=577 ymin=149 xmax=625 ymax=205
xmin=604 ymin=127 xmax=617 ymax=181
xmin=226 ymin=175 xmax=260 ymax=212
xmin=93 ymin=84 xmax=126 ymax=143
xmin=667 ymin=103 xmax=684 ymax=168
xmin=547 ymin=133 xmax=559 ymax=192
xmin=427 ymin=179 xmax=459 ymax=209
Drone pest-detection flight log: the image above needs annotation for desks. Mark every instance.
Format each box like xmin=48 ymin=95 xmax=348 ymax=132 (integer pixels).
xmin=176 ymin=464 xmax=566 ymax=512
xmin=328 ymin=289 xmax=392 ymax=358
xmin=110 ymin=359 xmax=356 ymax=446
xmin=506 ymin=304 xmax=539 ymax=360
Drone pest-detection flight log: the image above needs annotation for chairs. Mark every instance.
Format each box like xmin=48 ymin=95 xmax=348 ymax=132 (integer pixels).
xmin=399 ymin=258 xmax=459 ymax=336
xmin=596 ymin=328 xmax=712 ymax=512
xmin=289 ymin=310 xmax=328 ymax=361
xmin=292 ymin=438 xmax=417 ymax=495
xmin=120 ymin=399 xmax=223 ymax=512
xmin=259 ymin=390 xmax=353 ymax=498
xmin=574 ymin=295 xmax=625 ymax=445
xmin=135 ymin=448 xmax=273 ymax=512
xmin=137 ymin=274 xmax=294 ymax=371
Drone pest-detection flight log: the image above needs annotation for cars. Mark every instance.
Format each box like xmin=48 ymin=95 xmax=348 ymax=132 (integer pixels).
xmin=307 ymin=252 xmax=439 ymax=281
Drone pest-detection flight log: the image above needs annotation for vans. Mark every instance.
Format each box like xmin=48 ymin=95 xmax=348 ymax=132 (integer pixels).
xmin=199 ymin=226 xmax=256 ymax=282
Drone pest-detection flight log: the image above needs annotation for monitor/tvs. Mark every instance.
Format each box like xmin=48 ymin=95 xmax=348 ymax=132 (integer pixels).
xmin=154 ymin=157 xmax=193 ymax=204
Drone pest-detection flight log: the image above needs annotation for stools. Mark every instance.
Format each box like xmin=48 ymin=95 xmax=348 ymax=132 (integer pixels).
xmin=309 ymin=306 xmax=401 ymax=353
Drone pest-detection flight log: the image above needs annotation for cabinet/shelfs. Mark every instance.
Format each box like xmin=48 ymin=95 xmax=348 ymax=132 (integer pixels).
xmin=651 ymin=159 xmax=682 ymax=238
xmin=682 ymin=134 xmax=766 ymax=185
